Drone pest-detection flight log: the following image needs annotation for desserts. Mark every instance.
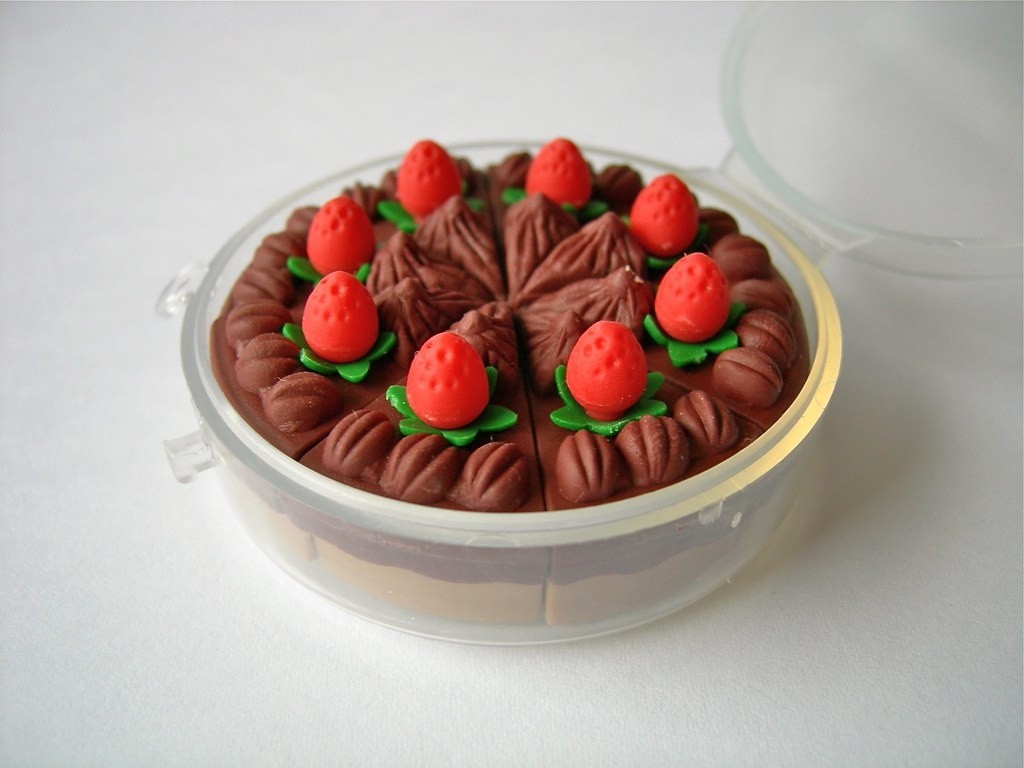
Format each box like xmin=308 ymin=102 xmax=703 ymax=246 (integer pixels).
xmin=206 ymin=139 xmax=814 ymax=630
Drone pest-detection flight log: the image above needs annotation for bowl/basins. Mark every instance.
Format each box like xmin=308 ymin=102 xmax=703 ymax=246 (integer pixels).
xmin=158 ymin=137 xmax=843 ymax=650
